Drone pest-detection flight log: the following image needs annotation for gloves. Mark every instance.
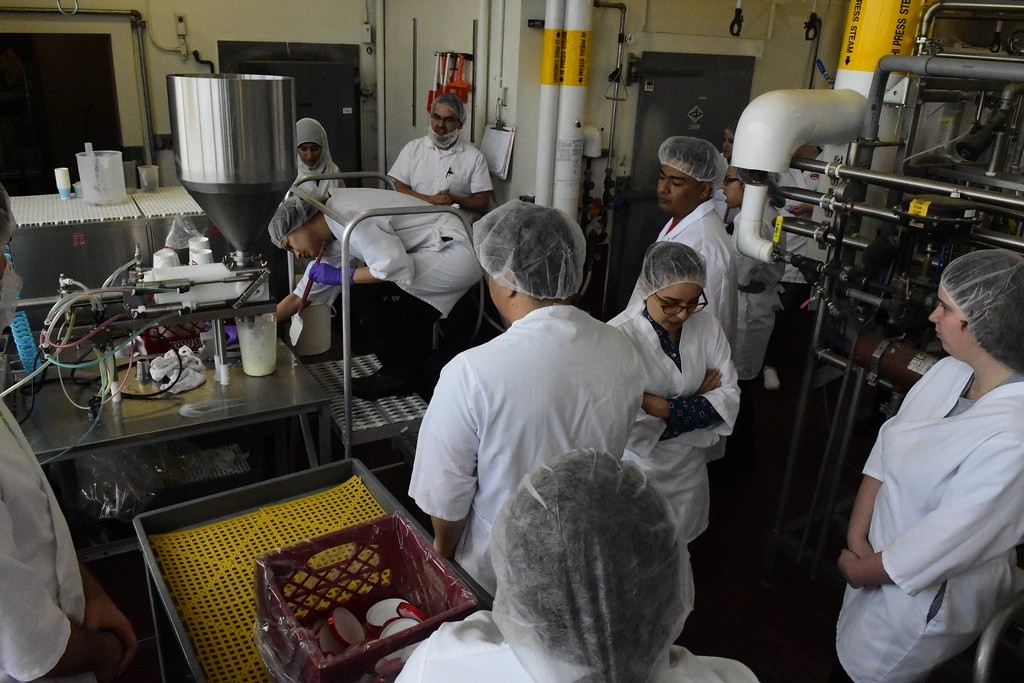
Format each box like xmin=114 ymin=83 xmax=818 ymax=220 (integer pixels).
xmin=308 ymin=263 xmax=356 ymax=285
xmin=205 ymin=321 xmax=237 ymax=346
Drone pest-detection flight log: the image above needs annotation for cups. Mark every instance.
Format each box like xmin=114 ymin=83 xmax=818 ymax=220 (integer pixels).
xmin=235 ymin=315 xmax=276 ymax=377
xmin=188 ymin=236 xmax=210 ymax=265
xmin=123 ymin=160 xmax=137 ymax=194
xmin=191 ymin=249 xmax=214 ymax=266
xmin=55 ymin=167 xmax=83 ymax=200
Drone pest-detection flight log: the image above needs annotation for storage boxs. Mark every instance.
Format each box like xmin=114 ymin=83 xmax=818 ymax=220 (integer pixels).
xmin=253 ymin=511 xmax=478 ymax=683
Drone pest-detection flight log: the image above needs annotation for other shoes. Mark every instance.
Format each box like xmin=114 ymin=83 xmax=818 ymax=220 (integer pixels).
xmin=351 ymin=366 xmax=418 ymax=398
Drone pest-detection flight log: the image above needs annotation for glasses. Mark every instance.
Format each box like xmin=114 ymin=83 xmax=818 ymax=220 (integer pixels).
xmin=651 ymin=284 xmax=709 ymax=313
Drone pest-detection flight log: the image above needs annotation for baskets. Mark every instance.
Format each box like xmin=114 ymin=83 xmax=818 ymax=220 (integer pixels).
xmin=140 ymin=321 xmax=211 ymax=357
xmin=254 ymin=513 xmax=478 ymax=683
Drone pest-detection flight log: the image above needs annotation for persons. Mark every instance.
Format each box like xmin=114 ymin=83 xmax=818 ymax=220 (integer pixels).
xmin=262 ymin=187 xmax=488 ymax=402
xmin=394 ymin=448 xmax=760 ymax=683
xmin=836 ymin=248 xmax=1024 ymax=683
xmin=720 ymin=117 xmax=833 ymax=484
xmin=408 ymin=197 xmax=651 ymax=598
xmin=598 ymin=241 xmax=741 ymax=557
xmin=626 ymin=138 xmax=738 ymax=553
xmin=391 ymin=93 xmax=495 ymax=218
xmin=292 ymin=118 xmax=353 ymax=191
xmin=0 ymin=183 xmax=139 ymax=683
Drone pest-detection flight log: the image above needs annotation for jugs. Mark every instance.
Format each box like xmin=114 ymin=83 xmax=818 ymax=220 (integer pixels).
xmin=75 ymin=142 xmax=127 ymax=207
xmin=138 ymin=164 xmax=159 ymax=192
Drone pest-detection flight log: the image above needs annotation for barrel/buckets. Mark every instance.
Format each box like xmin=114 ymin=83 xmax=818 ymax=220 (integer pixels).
xmin=294 ymin=273 xmax=332 ymax=354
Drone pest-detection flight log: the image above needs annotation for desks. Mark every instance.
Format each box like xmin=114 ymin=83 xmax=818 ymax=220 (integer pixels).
xmin=0 ymin=321 xmax=331 ymax=559
